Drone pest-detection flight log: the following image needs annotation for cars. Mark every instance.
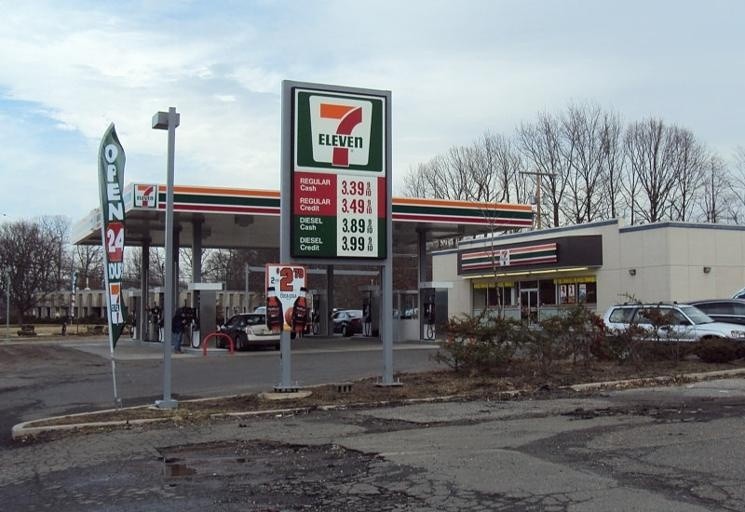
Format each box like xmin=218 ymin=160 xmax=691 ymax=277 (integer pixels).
xmin=600 ymin=287 xmax=745 ymax=359
xmin=331 ymin=308 xmax=363 ymax=337
xmin=216 ymin=306 xmax=281 ymax=352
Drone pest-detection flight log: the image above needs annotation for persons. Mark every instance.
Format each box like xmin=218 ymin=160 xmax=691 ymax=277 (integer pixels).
xmin=151 ymin=306 xmax=164 ymax=343
xmin=132 ymin=310 xmax=136 ymax=339
xmin=172 ymin=308 xmax=186 ymax=353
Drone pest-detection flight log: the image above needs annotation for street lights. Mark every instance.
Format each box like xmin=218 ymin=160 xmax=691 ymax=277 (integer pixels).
xmin=151 ymin=107 xmax=180 ymax=409
